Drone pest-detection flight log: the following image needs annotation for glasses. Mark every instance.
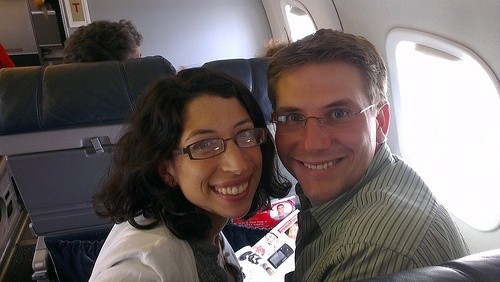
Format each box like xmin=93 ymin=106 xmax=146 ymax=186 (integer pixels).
xmin=267 ymin=104 xmax=377 ymax=132
xmin=166 ymin=127 xmax=265 ymax=161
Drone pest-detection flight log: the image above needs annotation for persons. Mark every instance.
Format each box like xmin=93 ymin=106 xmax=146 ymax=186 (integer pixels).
xmin=267 ymin=28 xmax=471 ymax=282
xmin=62 ymin=18 xmax=144 ymax=63
xmin=87 ymin=68 xmax=292 ymax=282
xmin=273 ymin=203 xmax=288 ymax=219
xmin=264 ymin=232 xmax=283 ymax=250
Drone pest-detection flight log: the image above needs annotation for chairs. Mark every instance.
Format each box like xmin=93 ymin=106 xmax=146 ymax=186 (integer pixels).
xmin=0 ymin=54 xmax=500 ymax=281
